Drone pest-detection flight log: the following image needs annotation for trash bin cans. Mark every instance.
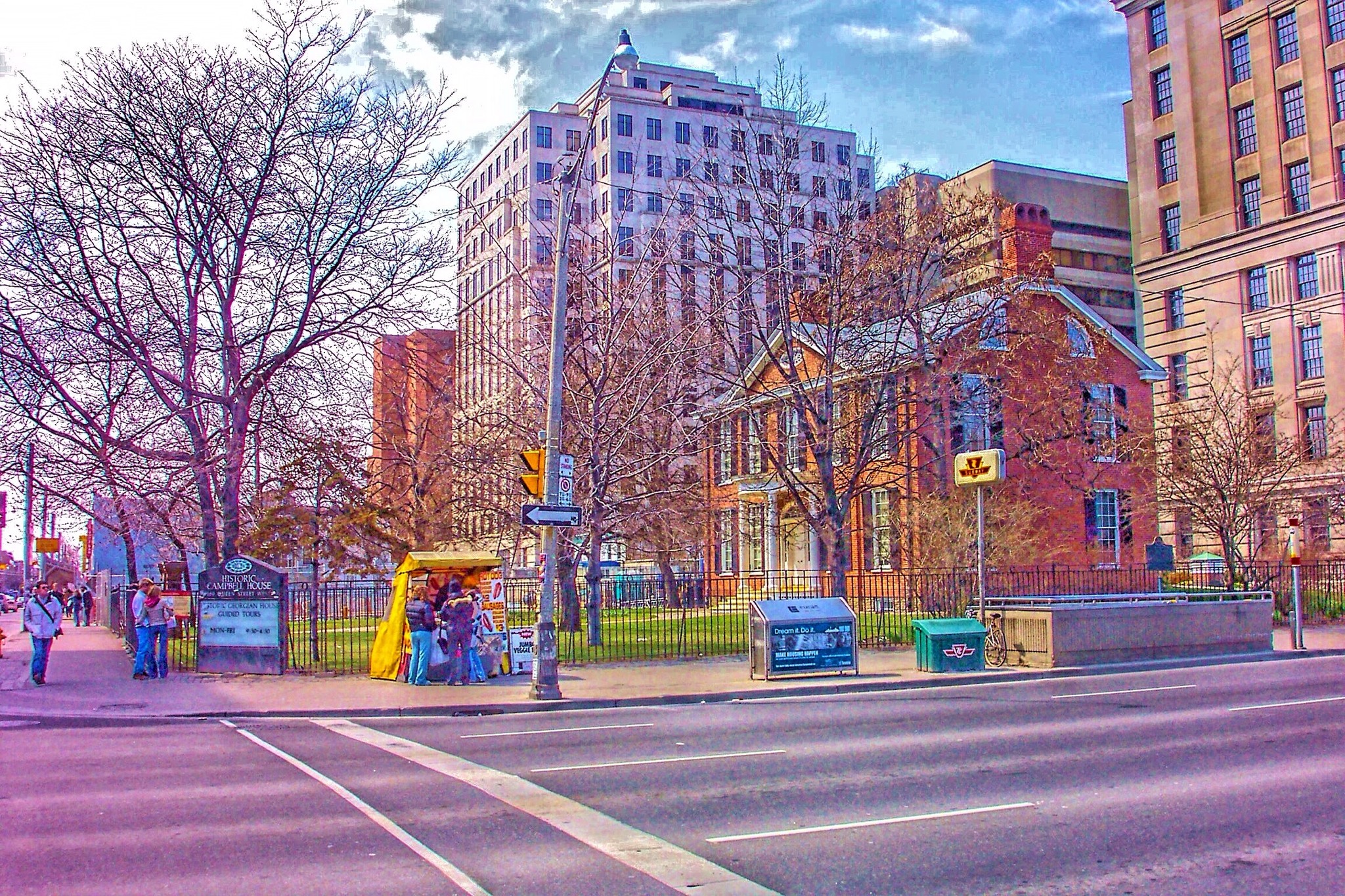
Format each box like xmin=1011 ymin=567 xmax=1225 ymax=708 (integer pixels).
xmin=911 ymin=618 xmax=988 ymax=672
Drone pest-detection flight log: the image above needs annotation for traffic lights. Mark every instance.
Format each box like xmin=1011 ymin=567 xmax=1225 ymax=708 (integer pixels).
xmin=519 ymin=449 xmax=545 ymax=498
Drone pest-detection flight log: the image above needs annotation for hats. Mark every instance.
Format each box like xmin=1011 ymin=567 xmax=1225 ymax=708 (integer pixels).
xmin=449 ymin=580 xmax=461 ymax=592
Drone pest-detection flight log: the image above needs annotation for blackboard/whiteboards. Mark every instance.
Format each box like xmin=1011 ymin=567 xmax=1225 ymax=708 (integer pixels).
xmin=510 ymin=628 xmax=534 ymax=663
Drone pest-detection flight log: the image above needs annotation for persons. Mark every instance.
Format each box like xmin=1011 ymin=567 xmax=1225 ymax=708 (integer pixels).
xmin=131 ymin=578 xmax=154 ymax=680
xmin=135 ymin=586 xmax=174 ymax=679
xmin=436 ymin=580 xmax=486 ymax=685
xmin=25 ymin=581 xmax=62 ymax=686
xmin=33 ymin=582 xmax=93 ymax=627
xmin=405 ymin=585 xmax=439 ymax=686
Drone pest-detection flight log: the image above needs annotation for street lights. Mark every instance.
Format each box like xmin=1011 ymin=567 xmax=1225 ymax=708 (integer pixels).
xmin=529 ymin=28 xmax=639 ymax=699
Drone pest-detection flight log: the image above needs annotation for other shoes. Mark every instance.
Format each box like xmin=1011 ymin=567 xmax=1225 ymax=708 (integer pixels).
xmin=134 ymin=674 xmax=148 ymax=679
xmin=37 ymin=678 xmax=46 ymax=686
xmin=143 ymin=672 xmax=149 ymax=676
xmin=34 ymin=674 xmax=42 ymax=682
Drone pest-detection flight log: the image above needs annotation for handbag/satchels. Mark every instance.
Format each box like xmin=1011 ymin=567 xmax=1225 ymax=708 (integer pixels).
xmin=167 ymin=615 xmax=177 ymax=629
xmin=437 ymin=638 xmax=449 ymax=654
xmin=54 ymin=627 xmax=64 ymax=639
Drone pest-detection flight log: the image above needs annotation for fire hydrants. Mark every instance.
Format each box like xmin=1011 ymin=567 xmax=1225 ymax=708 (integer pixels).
xmin=0 ymin=627 xmax=7 ymax=658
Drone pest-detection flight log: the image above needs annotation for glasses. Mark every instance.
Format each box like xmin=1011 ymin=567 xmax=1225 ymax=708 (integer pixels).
xmin=38 ymin=586 xmax=49 ymax=590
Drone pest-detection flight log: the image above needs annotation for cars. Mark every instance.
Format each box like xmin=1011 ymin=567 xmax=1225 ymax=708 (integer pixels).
xmin=5 ymin=595 xmax=18 ymax=612
xmin=0 ymin=594 xmax=10 ymax=613
xmin=16 ymin=597 xmax=24 ymax=608
